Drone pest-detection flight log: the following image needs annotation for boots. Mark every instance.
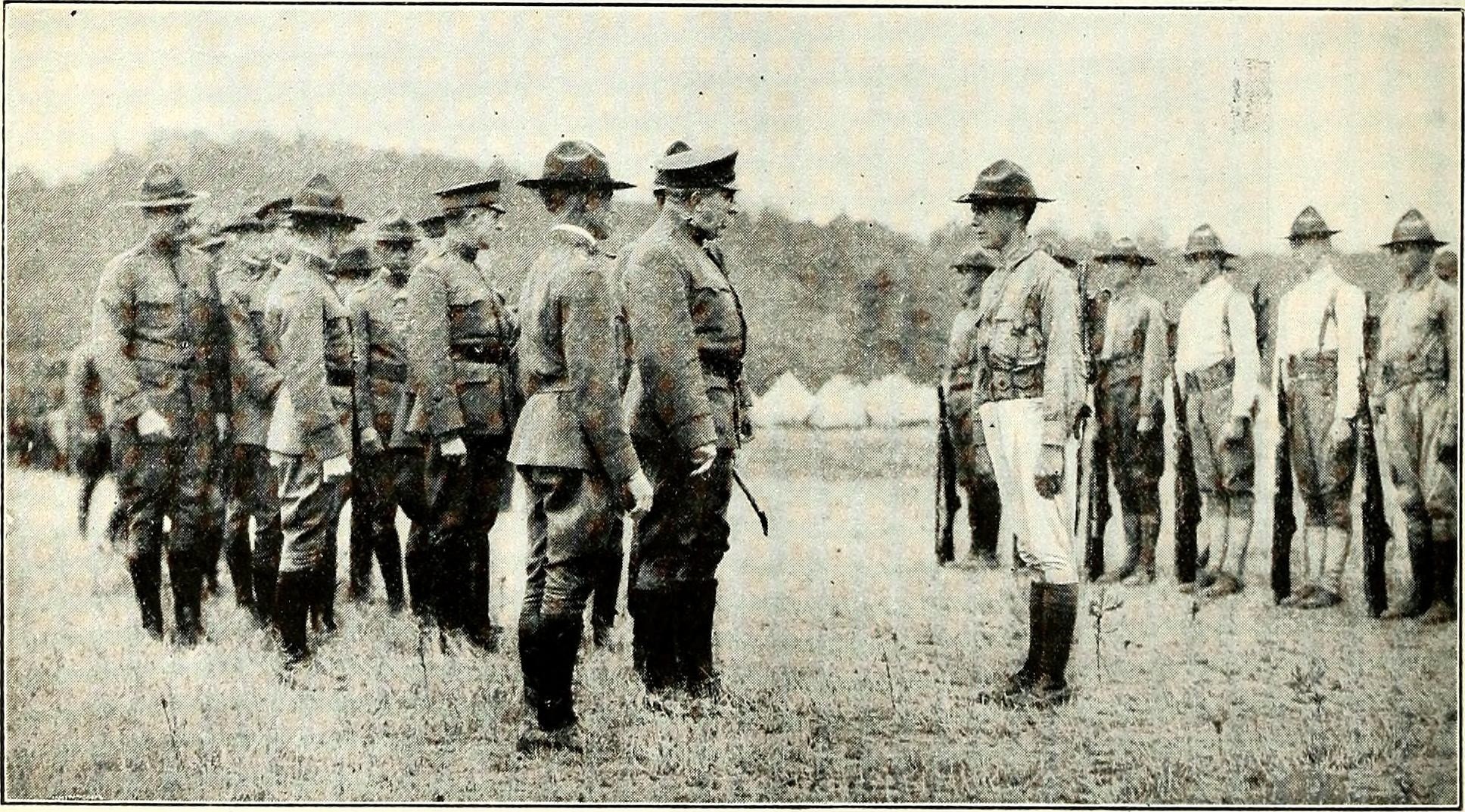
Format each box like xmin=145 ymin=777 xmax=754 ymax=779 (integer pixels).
xmin=980 ymin=583 xmax=1079 ymax=710
xmin=125 ymin=555 xmax=721 ymax=753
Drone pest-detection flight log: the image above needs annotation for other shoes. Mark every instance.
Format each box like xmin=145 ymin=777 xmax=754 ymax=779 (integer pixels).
xmin=1422 ymin=602 xmax=1458 ymax=626
xmin=1120 ymin=565 xmax=1151 ymax=586
xmin=1097 ymin=565 xmax=1135 ymax=583
xmin=1379 ymin=596 xmax=1430 ymax=620
xmin=1208 ymin=574 xmax=1240 ymax=603
xmin=1299 ymin=591 xmax=1338 ymax=610
xmin=1180 ymin=573 xmax=1219 ymax=595
xmin=947 ymin=551 xmax=998 ymax=567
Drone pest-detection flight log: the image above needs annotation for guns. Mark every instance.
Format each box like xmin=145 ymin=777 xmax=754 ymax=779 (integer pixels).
xmin=1077 ymin=259 xmax=1100 ymax=570
xmin=1272 ymin=358 xmax=1296 ymax=604
xmin=1174 ymin=364 xmax=1199 ymax=584
xmin=1352 ymin=291 xmax=1390 ymax=621
xmin=933 ymin=382 xmax=959 ymax=565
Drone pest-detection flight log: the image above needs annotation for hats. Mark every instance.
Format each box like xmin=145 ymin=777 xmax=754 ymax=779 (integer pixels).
xmin=657 ymin=144 xmax=740 ymax=193
xmin=1180 ymin=224 xmax=1238 ymax=257
xmin=126 ymin=162 xmax=507 ymax=275
xmin=517 ymin=141 xmax=635 ymax=189
xmin=1046 ymin=245 xmax=1077 ymax=265
xmin=952 ymin=250 xmax=996 ymax=273
xmin=1378 ymin=209 xmax=1449 ymax=249
xmin=1093 ymin=237 xmax=1156 ymax=266
xmin=1285 ymin=208 xmax=1341 ymax=240
xmin=954 ymin=159 xmax=1054 ymax=202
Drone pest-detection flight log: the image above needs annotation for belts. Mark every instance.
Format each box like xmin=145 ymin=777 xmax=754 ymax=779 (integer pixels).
xmin=368 ymin=361 xmax=408 ymax=384
xmin=462 ymin=346 xmax=512 ymax=367
xmin=328 ymin=369 xmax=353 ymax=388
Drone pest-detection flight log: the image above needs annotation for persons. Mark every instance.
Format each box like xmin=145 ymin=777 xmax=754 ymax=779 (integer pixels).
xmin=67 ymin=194 xmax=423 ymax=619
xmin=1173 ymin=224 xmax=1257 ymax=600
xmin=951 ymin=159 xmax=1089 ymax=710
xmin=1092 ymin=236 xmax=1172 ymax=585
xmin=404 ymin=178 xmax=517 ymax=652
xmin=622 ymin=141 xmax=756 ymax=704
xmin=506 ymin=141 xmax=653 ymax=754
xmin=264 ymin=173 xmax=365 ymax=663
xmin=1272 ymin=204 xmax=1364 ymax=609
xmin=1030 ymin=230 xmax=1076 ymax=278
xmin=1370 ymin=209 xmax=1460 ymax=620
xmin=91 ymin=160 xmax=228 ymax=647
xmin=938 ymin=246 xmax=1002 ymax=562
